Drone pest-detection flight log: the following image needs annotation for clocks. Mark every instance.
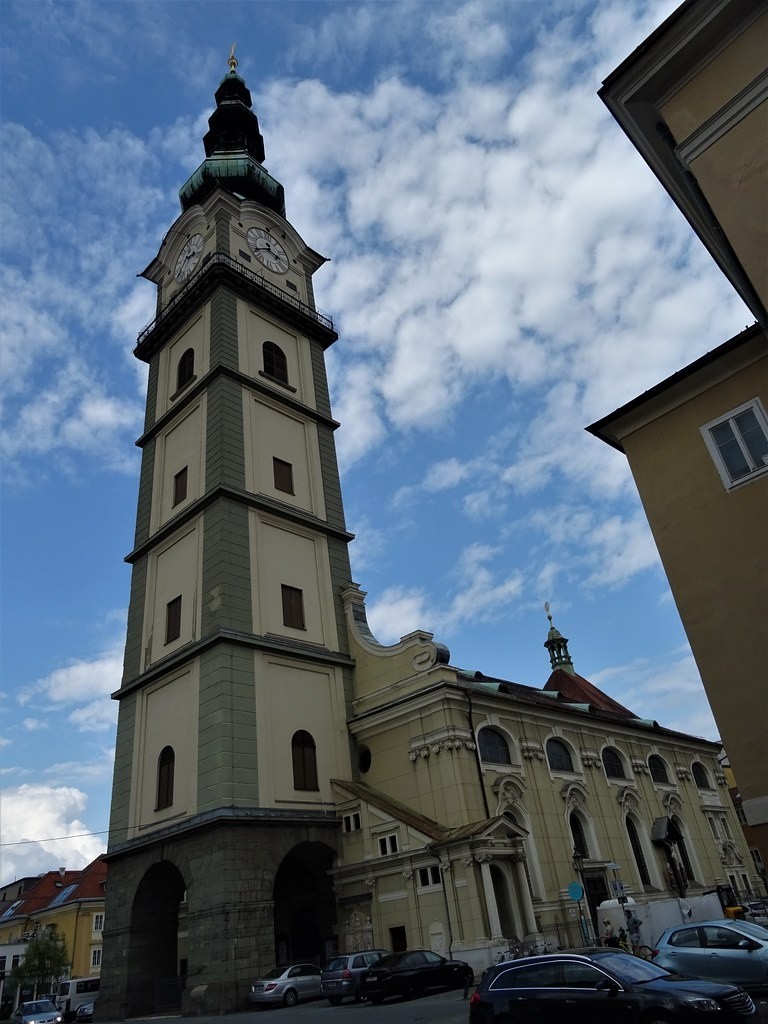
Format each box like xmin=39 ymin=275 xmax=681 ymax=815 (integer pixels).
xmin=247 ymin=227 xmax=290 ymax=274
xmin=173 ymin=232 xmax=203 ymax=282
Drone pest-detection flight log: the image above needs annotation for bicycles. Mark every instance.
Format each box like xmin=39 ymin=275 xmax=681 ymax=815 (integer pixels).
xmin=620 ymin=937 xmax=653 ymax=961
xmin=481 ymin=936 xmax=620 ymax=987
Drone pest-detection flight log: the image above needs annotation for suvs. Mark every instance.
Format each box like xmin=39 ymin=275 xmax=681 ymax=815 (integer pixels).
xmin=469 ymin=918 xmax=768 ymax=1024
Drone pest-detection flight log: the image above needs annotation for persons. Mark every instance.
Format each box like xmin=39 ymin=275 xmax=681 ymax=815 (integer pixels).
xmin=600 ymin=918 xmax=620 ymax=948
xmin=623 ymin=909 xmax=642 ymax=955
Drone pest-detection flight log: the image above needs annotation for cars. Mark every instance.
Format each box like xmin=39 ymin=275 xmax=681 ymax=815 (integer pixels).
xmin=248 ymin=962 xmax=323 ymax=1007
xmin=321 ymin=948 xmax=473 ymax=1005
xmin=737 ymin=901 xmax=768 ymax=925
xmin=9 ymin=977 xmax=100 ymax=1024
